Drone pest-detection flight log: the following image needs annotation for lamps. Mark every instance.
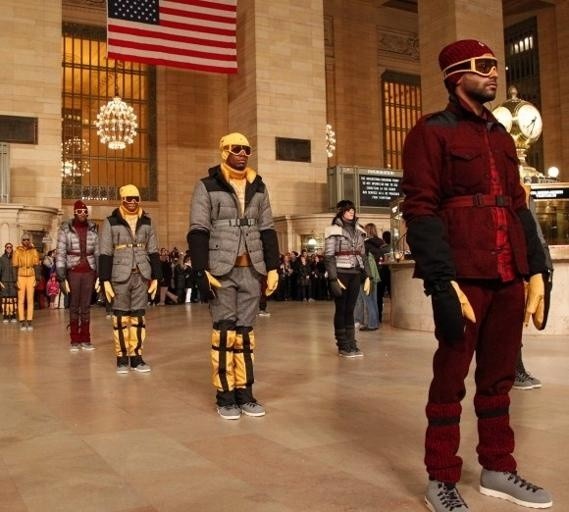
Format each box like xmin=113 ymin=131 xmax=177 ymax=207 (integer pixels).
xmin=326 ymin=125 xmax=335 ymax=158
xmin=61 ymin=137 xmax=89 ymax=181
xmin=94 ymin=59 xmax=138 ymax=149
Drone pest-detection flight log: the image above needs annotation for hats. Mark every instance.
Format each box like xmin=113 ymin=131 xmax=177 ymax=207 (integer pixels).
xmin=74 ymin=200 xmax=87 ymax=210
xmin=21 ymin=234 xmax=30 ymax=240
xmin=438 ymin=39 xmax=496 ymax=95
xmin=337 ymin=199 xmax=356 ymax=213
xmin=119 ymin=184 xmax=140 ymax=197
xmin=218 ymin=132 xmax=250 ymax=162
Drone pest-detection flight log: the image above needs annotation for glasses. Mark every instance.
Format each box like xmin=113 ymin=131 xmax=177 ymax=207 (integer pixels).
xmin=23 ymin=239 xmax=29 ymax=241
xmin=221 ymin=144 xmax=252 ymax=157
xmin=7 ymin=247 xmax=12 ymax=249
xmin=440 ymin=56 xmax=498 ymax=80
xmin=122 ymin=196 xmax=140 ymax=203
xmin=73 ymin=209 xmax=88 ymax=216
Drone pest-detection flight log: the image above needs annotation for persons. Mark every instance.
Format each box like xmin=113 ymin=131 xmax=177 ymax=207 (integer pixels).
xmin=56 ymin=199 xmax=100 ymax=352
xmin=325 ymin=200 xmax=372 ymax=359
xmin=1 ymin=234 xmax=70 ymax=330
xmin=187 ymin=132 xmax=280 ymax=421
xmin=401 ymin=38 xmax=554 ymax=511
xmin=512 ymin=160 xmax=554 ymax=389
xmin=353 ymin=223 xmax=390 ymax=331
xmin=99 ymin=184 xmax=162 ymax=374
xmin=256 ymin=249 xmax=334 ymax=317
xmin=90 ymin=246 xmax=209 ymax=321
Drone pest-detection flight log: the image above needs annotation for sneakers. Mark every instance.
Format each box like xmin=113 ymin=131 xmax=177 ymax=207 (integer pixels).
xmin=3 ymin=315 xmax=266 ymax=422
xmin=521 ymin=364 xmax=542 ymax=388
xmin=257 ymin=310 xmax=364 ymax=358
xmin=355 ymin=322 xmax=378 ymax=331
xmin=512 ymin=370 xmax=533 ymax=390
xmin=424 ymin=479 xmax=472 ymax=512
xmin=480 ymin=468 xmax=552 ymax=509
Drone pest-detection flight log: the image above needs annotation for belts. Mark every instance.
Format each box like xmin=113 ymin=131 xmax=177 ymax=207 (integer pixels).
xmin=20 ymin=266 xmax=34 ymax=268
xmin=336 ymin=251 xmax=361 ymax=256
xmin=441 ymin=193 xmax=513 ymax=209
xmin=234 ymin=255 xmax=251 ymax=268
xmin=114 ymin=243 xmax=145 ymax=249
xmin=131 ymin=269 xmax=140 ymax=274
xmin=67 ymin=251 xmax=93 ymax=256
xmin=211 ymin=219 xmax=257 ymax=227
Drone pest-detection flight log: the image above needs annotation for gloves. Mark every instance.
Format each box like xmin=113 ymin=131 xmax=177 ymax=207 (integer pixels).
xmin=0 ymin=280 xmax=39 ymax=289
xmin=194 ymin=269 xmax=221 ymax=305
xmin=147 ymin=279 xmax=158 ymax=300
xmin=329 ymin=278 xmax=347 ymax=297
xmin=59 ymin=279 xmax=71 ymax=296
xmin=423 ymin=279 xmax=477 ymax=352
xmin=265 ymin=269 xmax=279 ymax=296
xmin=363 ymin=278 xmax=371 ymax=296
xmin=524 ymin=270 xmax=553 ymax=330
xmin=94 ymin=277 xmax=102 ymax=294
xmin=103 ymin=281 xmax=115 ymax=304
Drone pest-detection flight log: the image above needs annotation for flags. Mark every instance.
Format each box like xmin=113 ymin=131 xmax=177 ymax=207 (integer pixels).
xmin=107 ymin=0 xmax=238 ymax=77
